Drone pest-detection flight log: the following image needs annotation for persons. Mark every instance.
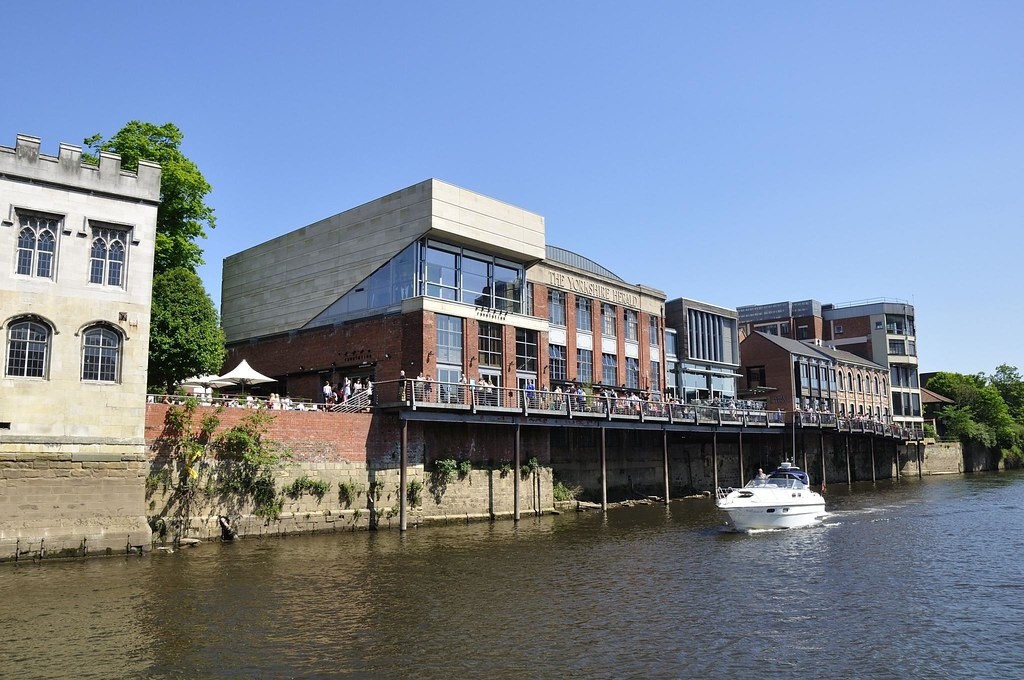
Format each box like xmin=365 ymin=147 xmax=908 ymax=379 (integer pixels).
xmin=754 ymin=468 xmax=768 ymax=485
xmin=796 ymin=404 xmax=913 ymax=437
xmin=159 ymin=391 xmax=306 ymax=410
xmin=399 ymin=369 xmax=781 ymax=424
xmin=322 ymin=376 xmax=373 ymax=411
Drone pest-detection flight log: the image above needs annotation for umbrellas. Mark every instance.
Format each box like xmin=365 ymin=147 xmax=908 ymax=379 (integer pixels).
xmin=178 ymin=358 xmax=279 ymax=395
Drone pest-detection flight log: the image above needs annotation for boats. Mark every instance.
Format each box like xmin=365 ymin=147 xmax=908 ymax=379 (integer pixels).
xmin=715 ymin=462 xmax=826 ymax=531
